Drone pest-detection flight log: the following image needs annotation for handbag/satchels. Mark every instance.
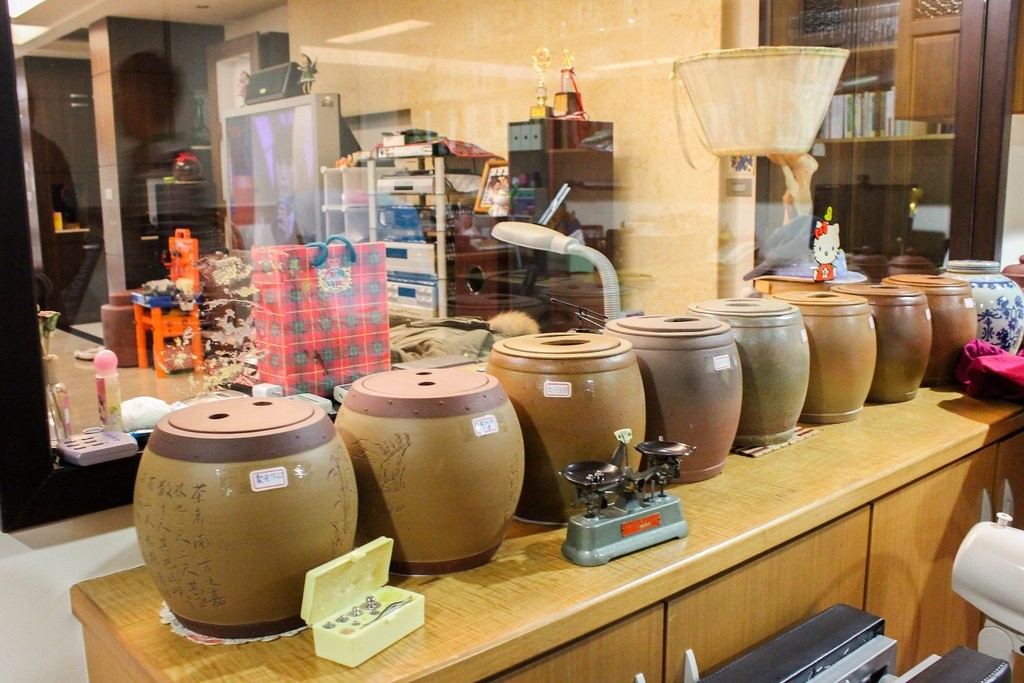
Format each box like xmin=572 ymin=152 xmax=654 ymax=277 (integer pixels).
xmin=254 ymin=235 xmax=391 ymax=399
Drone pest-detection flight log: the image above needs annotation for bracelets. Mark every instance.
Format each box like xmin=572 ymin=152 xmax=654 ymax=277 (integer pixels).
xmin=33 ymin=272 xmax=55 ymax=299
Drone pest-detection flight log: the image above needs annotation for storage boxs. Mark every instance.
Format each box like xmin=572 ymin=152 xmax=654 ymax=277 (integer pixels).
xmin=300 ymin=537 xmax=425 ymax=668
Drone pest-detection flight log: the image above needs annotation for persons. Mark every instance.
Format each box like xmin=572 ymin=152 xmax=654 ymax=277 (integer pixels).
xmin=115 ymin=48 xmax=223 ymax=288
xmin=297 ymin=53 xmax=318 ymax=94
xmin=485 ymin=176 xmax=510 ymax=206
xmin=235 ymin=71 xmax=250 ymax=107
xmin=23 ymin=89 xmax=89 ymax=335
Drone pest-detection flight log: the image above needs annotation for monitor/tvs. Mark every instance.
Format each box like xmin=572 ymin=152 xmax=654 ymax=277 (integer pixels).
xmin=221 ymin=93 xmax=362 ymax=254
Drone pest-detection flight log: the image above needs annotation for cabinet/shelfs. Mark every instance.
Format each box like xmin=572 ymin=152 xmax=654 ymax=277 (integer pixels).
xmin=507 ymin=115 xmax=616 ymax=329
xmin=770 ymin=0 xmax=960 ymax=183
xmin=366 ymin=156 xmax=486 ymax=319
xmin=70 ymin=384 xmax=1024 ymax=683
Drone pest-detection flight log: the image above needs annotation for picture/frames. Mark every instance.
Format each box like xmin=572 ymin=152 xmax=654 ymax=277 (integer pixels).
xmin=474 ymin=161 xmax=509 ymax=213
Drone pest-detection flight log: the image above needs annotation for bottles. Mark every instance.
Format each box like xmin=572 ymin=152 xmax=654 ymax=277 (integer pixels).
xmin=42 ymin=354 xmax=73 ymax=449
xmin=93 ymin=350 xmax=122 ymax=432
xmin=191 ymin=99 xmax=210 ymax=146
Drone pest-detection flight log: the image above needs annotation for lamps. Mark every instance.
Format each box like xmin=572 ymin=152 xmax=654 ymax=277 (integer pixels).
xmin=492 ymin=221 xmax=619 ymax=324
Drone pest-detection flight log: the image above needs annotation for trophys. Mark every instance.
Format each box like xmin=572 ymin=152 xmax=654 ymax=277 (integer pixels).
xmin=530 ymin=45 xmax=585 ymax=119
